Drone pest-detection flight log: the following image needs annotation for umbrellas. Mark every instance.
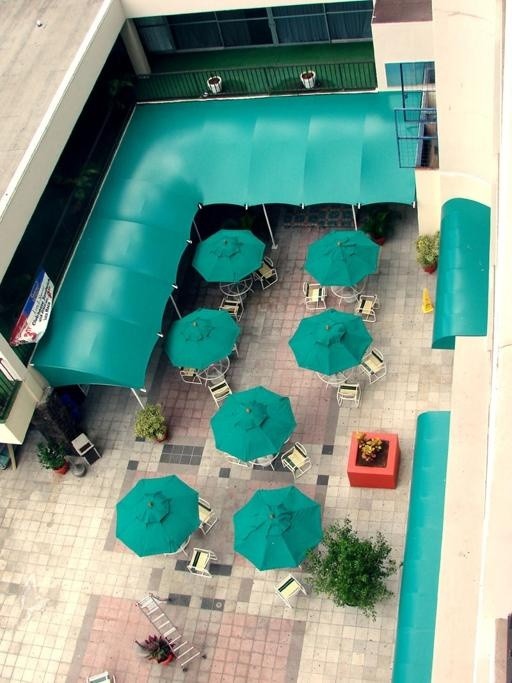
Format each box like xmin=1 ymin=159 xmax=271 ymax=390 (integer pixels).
xmin=303 ymin=227 xmax=384 ymax=288
xmin=190 ymin=226 xmax=268 ymax=284
xmin=285 ymin=307 xmax=375 ymax=377
xmin=231 ymin=482 xmax=326 ymax=573
xmin=208 ymin=383 xmax=298 ymax=463
xmin=162 ymin=304 xmax=242 ymax=370
xmin=111 ymin=473 xmax=205 ymax=558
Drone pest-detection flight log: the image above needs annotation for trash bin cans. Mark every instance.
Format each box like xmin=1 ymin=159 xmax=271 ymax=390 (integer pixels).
xmin=71 ymin=432 xmax=101 ymax=466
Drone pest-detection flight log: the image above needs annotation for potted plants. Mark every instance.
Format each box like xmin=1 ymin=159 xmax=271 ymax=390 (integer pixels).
xmin=145 ymin=635 xmax=175 ymax=664
xmin=362 ymin=213 xmax=387 ymax=245
xmin=134 ymin=404 xmax=168 ymax=443
xmin=414 ymin=230 xmax=440 ymax=273
xmin=347 ymin=431 xmax=400 ymax=488
xmin=37 ymin=442 xmax=68 ymax=475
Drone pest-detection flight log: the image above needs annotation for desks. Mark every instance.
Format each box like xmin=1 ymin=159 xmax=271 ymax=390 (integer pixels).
xmin=219 ymin=274 xmax=254 ymax=301
xmin=331 ymin=281 xmax=365 ymax=307
xmin=248 ymin=452 xmax=279 ymax=471
xmin=164 ymin=536 xmax=190 ymax=558
xmin=197 ymin=357 xmax=230 ymax=387
xmin=317 ymin=369 xmax=353 ymax=390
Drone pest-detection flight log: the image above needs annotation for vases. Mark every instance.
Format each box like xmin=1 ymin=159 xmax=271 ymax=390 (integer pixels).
xmin=300 ymin=71 xmax=316 ymax=88
xmin=207 ymin=76 xmax=222 ymax=94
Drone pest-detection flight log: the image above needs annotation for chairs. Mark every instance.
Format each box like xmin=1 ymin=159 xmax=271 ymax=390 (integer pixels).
xmin=208 ymin=379 xmax=233 ymax=408
xmin=274 ymin=573 xmax=307 ymax=608
xmin=281 ymin=442 xmax=311 ymax=480
xmin=358 ymin=348 xmax=385 ymax=384
xmin=232 ymin=344 xmax=238 ymax=356
xmin=198 ymin=498 xmax=217 ymax=535
xmin=303 ymin=281 xmax=326 ymax=310
xmin=187 ymin=547 xmax=217 ymax=578
xmin=218 ymin=299 xmax=244 ymax=321
xmin=337 ymin=383 xmax=361 ymax=407
xmin=180 ymin=368 xmax=202 ymax=385
xmin=355 ymin=295 xmax=380 ymax=323
xmin=253 ymin=256 xmax=278 ymax=290
xmin=225 ymin=453 xmax=248 ymax=467
xmin=87 ymin=670 xmax=115 ymax=683
